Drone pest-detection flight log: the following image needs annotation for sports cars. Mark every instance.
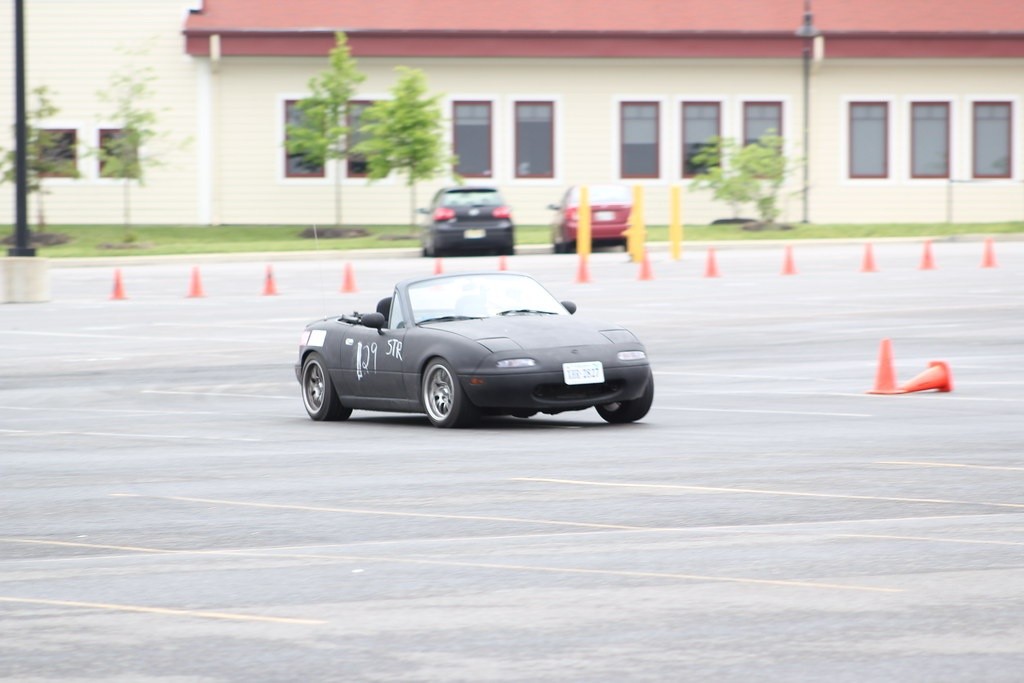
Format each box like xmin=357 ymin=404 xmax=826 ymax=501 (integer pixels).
xmin=294 ymin=272 xmax=654 ymax=429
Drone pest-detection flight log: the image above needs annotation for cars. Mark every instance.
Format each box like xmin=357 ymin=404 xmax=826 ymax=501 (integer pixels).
xmin=419 ymin=187 xmax=514 ymax=258
xmin=546 ymin=185 xmax=635 ymax=253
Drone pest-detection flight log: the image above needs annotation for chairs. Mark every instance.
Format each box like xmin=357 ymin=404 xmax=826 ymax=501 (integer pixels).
xmin=376 ymin=297 xmax=412 ymax=328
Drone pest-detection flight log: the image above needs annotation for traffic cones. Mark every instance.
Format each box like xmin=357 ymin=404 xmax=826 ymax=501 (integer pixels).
xmin=261 ymin=264 xmax=279 ymax=294
xmin=916 ymin=240 xmax=935 ymax=270
xmin=574 ymin=255 xmax=589 ymax=282
xmin=866 ymin=339 xmax=953 ymax=395
xmin=782 ymin=245 xmax=796 ymax=274
xmin=186 ymin=267 xmax=203 ymax=298
xmin=340 ymin=263 xmax=355 ymax=291
xmin=108 ymin=267 xmax=129 ymax=300
xmin=861 ymin=243 xmax=877 ymax=271
xmin=981 ymin=239 xmax=997 ymax=269
xmin=704 ymin=246 xmax=718 ymax=278
xmin=637 ymin=246 xmax=655 ymax=280
